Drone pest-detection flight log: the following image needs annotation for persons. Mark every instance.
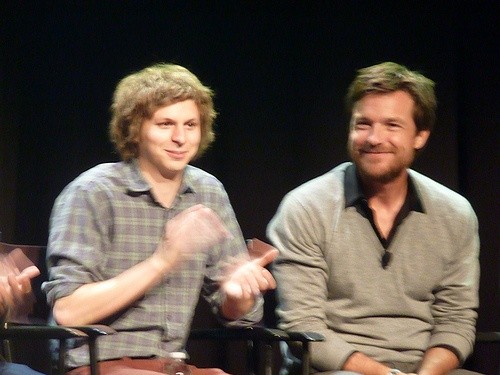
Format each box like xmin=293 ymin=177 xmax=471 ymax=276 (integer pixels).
xmin=42 ymin=65 xmax=278 ymax=375
xmin=0 ymin=248 xmax=42 ymax=375
xmin=266 ymin=62 xmax=484 ymax=375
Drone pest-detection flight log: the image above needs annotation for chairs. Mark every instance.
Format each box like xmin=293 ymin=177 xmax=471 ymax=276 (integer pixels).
xmin=0 ymin=238 xmax=326 ymax=375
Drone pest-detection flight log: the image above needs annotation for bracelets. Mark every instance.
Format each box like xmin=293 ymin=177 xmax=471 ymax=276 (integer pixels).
xmin=389 ymin=369 xmax=399 ymax=375
